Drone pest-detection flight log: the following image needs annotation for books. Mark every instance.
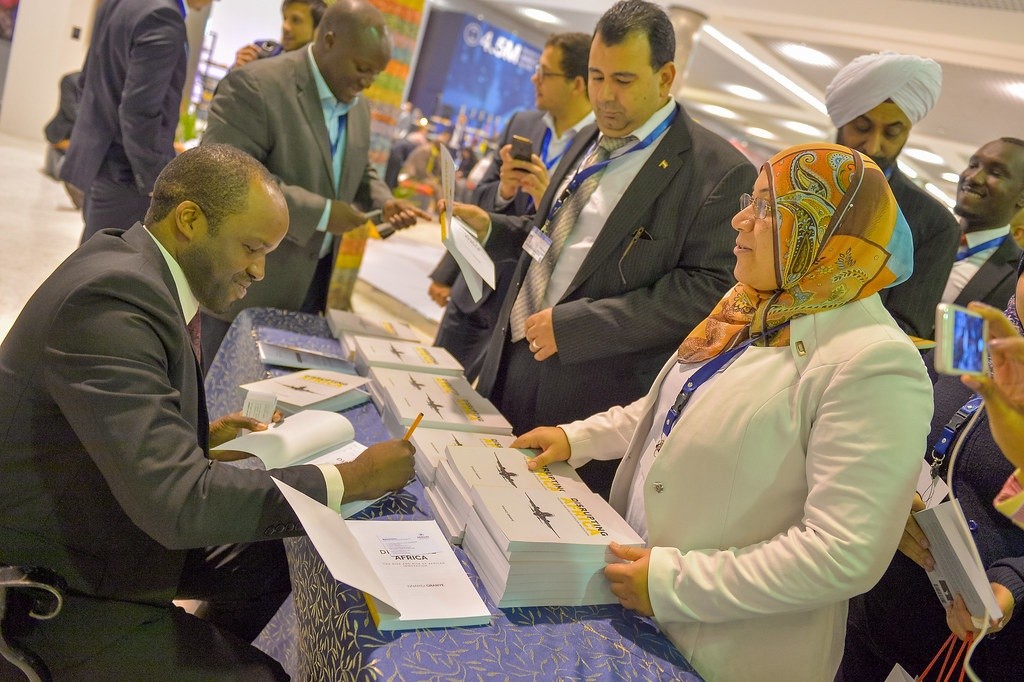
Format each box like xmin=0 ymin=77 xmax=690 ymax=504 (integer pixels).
xmin=270 ymin=475 xmax=491 ymax=631
xmin=212 ymin=409 xmax=392 ymax=520
xmin=240 ymin=368 xmax=372 ymax=419
xmin=913 ymin=498 xmax=1004 ymax=621
xmin=326 ymin=309 xmax=646 ymax=608
xmin=258 ymin=340 xmax=357 ymax=374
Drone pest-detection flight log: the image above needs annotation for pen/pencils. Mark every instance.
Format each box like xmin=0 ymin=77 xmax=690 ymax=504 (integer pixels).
xmin=402 ymin=413 xmax=424 ymax=440
xmin=364 ymin=209 xmax=383 ymax=218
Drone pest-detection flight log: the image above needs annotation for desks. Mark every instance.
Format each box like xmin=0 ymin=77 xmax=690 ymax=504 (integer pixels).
xmin=205 ymin=309 xmax=705 ymax=682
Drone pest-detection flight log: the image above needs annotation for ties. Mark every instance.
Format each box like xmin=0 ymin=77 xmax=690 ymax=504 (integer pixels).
xmin=958 ymin=233 xmax=969 ymax=247
xmin=186 ymin=306 xmax=202 ymax=364
xmin=509 ymin=135 xmax=638 ymax=342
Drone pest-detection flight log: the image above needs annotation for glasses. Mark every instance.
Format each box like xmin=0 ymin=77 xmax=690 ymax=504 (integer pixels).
xmin=533 ymin=64 xmax=577 ymax=85
xmin=739 ymin=194 xmax=773 ymax=220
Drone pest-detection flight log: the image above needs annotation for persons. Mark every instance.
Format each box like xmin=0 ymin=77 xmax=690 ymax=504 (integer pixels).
xmin=385 ymin=131 xmax=477 ymax=190
xmin=202 ymin=0 xmax=432 ymax=378
xmin=46 ymin=71 xmax=86 ymax=144
xmin=833 ymin=137 xmax=1024 ymax=682
xmin=824 ymin=51 xmax=960 ymax=354
xmin=65 ymin=0 xmax=216 ymax=243
xmin=226 ymin=0 xmax=327 ymax=74
xmin=512 ymin=143 xmax=933 ymax=682
xmin=428 ymin=0 xmax=758 ymax=504
xmin=0 ymin=143 xmax=417 ymax=682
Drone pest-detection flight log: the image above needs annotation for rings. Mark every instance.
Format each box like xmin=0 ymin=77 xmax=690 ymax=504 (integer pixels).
xmin=532 ymin=339 xmax=540 ymax=348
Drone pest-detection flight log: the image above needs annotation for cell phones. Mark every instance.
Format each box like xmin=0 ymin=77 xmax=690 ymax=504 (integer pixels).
xmin=510 ymin=135 xmax=533 ymax=174
xmin=933 ymin=302 xmax=990 ymax=375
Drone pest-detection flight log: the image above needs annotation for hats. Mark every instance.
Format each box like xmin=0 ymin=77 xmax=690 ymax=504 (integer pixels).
xmin=825 ymin=50 xmax=945 ymax=128
